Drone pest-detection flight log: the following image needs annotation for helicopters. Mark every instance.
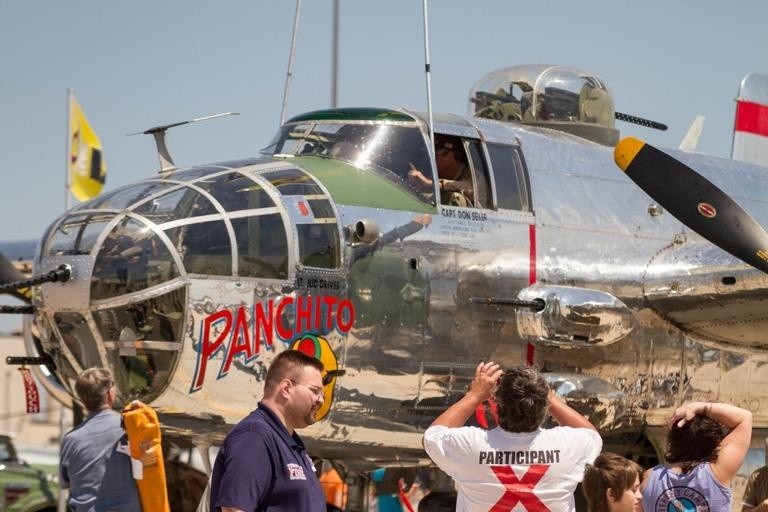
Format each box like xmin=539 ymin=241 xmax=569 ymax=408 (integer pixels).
xmin=0 ymin=2 xmax=767 ymax=487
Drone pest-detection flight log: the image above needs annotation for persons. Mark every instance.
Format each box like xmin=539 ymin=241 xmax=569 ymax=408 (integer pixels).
xmin=420 ymin=359 xmax=599 ymax=511
xmin=741 ymin=439 xmax=768 ymax=512
xmin=632 ymin=399 xmax=753 ymax=512
xmin=579 ymin=453 xmax=645 ymax=512
xmin=405 ymin=138 xmax=488 ymax=207
xmin=57 ymin=365 xmax=144 ymax=511
xmin=194 ymin=347 xmax=333 ymax=512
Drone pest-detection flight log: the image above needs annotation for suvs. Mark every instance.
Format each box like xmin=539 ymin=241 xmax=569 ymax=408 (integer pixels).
xmin=0 ymin=432 xmax=62 ymax=512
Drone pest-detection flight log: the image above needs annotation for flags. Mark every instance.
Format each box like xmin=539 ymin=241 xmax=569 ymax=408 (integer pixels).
xmin=68 ymin=94 xmax=108 ymax=207
